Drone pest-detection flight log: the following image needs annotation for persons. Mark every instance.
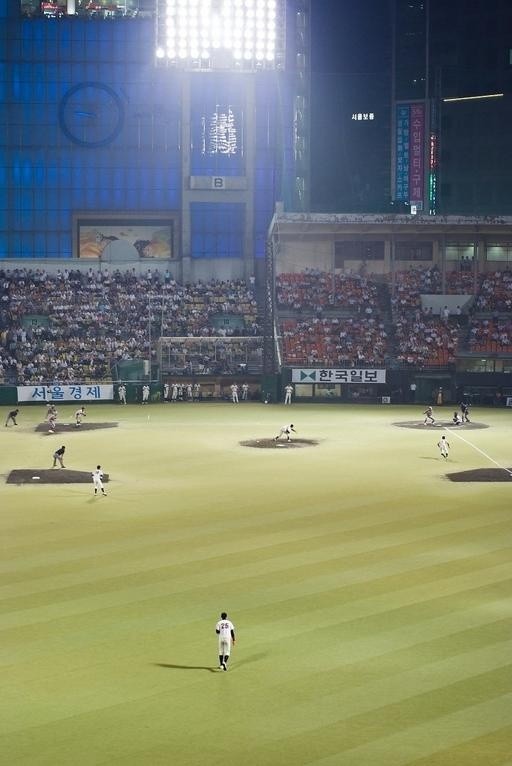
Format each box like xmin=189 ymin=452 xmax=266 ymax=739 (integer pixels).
xmin=91 ymin=465 xmax=108 ymax=496
xmin=6 ymin=409 xmax=19 ymax=426
xmin=79 ymin=236 xmax=120 ymax=259
xmin=52 ymin=445 xmax=65 ymax=469
xmin=133 ymin=238 xmax=172 ymax=258
xmin=46 ymin=405 xmax=87 ymax=433
xmin=273 ymin=423 xmax=299 ymax=442
xmin=215 ymin=611 xmax=236 ymax=672
xmin=1 ymin=256 xmax=512 ymax=387
xmin=118 ymin=382 xmax=293 ymax=405
xmin=437 ymin=436 xmax=451 ymax=460
xmin=423 ymin=402 xmax=472 ymax=425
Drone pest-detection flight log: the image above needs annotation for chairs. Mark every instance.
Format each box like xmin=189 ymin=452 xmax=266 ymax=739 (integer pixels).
xmin=0 ymin=270 xmax=512 ymax=386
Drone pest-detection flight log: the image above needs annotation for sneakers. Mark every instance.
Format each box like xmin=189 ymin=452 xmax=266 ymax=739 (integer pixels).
xmin=219 ymin=662 xmax=227 ymax=671
